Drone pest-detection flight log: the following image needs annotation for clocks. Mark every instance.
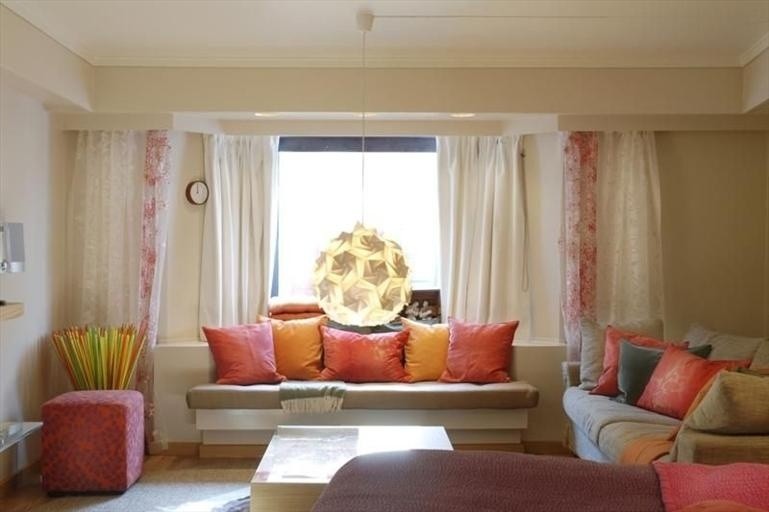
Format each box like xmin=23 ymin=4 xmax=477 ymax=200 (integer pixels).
xmin=186 ymin=178 xmax=209 ymax=206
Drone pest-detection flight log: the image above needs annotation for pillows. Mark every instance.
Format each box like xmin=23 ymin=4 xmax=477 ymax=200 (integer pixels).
xmin=437 ymin=315 xmax=520 ymax=382
xmin=684 ymin=371 xmax=769 ymax=435
xmin=637 ymin=343 xmax=752 ymax=420
xmin=610 ymin=340 xmax=712 ymax=405
xmin=202 ymin=320 xmax=287 ymax=383
xmin=589 ymin=325 xmax=689 ymax=395
xmin=320 ymin=325 xmax=409 ymax=382
xmin=257 ymin=315 xmax=328 ymax=380
xmin=579 ymin=317 xmax=663 ymax=389
xmin=401 ymin=318 xmax=449 ymax=383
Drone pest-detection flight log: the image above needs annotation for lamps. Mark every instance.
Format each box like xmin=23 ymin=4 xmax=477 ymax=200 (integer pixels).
xmin=312 ymin=14 xmax=413 ymax=328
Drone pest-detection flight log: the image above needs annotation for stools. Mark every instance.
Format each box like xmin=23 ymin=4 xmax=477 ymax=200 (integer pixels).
xmin=42 ymin=391 xmax=144 ymax=494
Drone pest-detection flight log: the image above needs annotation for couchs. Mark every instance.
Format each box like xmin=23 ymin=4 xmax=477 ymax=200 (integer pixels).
xmin=562 ymin=323 xmax=769 ymax=464
xmin=311 ymin=450 xmax=768 ymax=511
xmin=186 ymin=380 xmax=539 ymax=459
